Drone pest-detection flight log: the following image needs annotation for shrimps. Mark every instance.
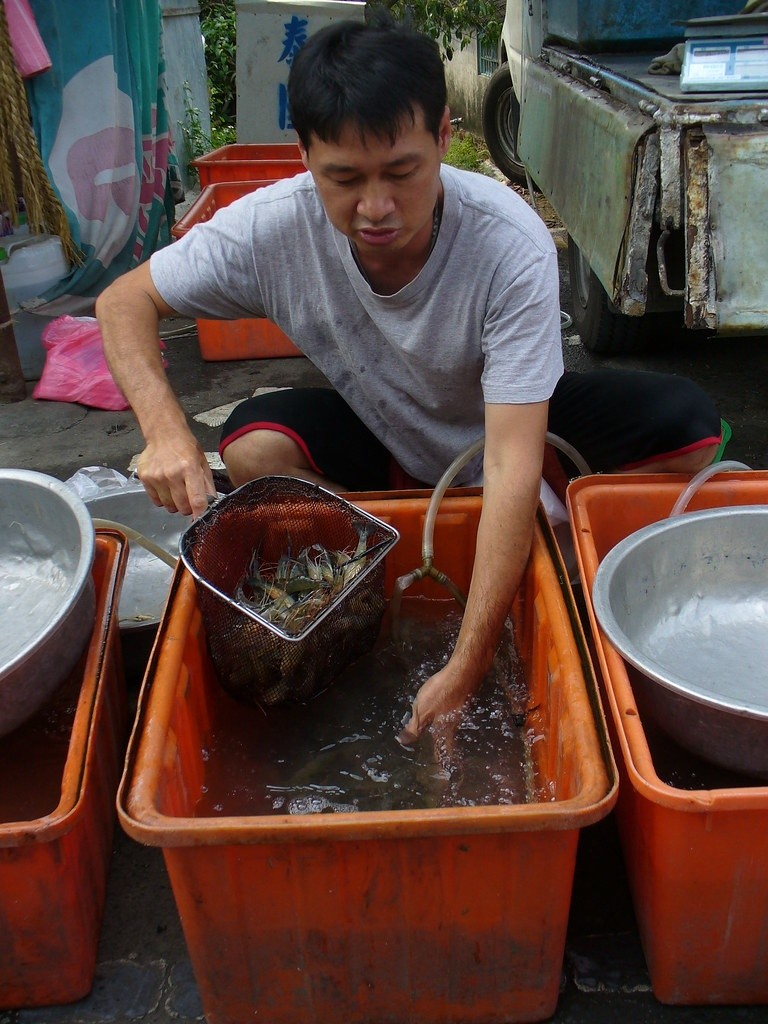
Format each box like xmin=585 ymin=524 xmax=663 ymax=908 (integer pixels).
xmin=212 ymin=520 xmax=380 ymax=641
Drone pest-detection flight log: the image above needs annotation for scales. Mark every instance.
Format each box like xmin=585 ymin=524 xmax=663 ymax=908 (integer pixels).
xmin=670 ymin=11 xmax=768 ymax=93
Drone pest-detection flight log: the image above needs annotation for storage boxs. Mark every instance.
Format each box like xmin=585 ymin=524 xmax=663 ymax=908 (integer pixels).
xmin=188 ymin=143 xmax=307 ymax=195
xmin=566 ymin=470 xmax=768 ymax=1004
xmin=0 ymin=524 xmax=130 ymax=1009
xmin=113 ymin=483 xmax=625 ymax=1024
xmin=169 ymin=178 xmax=305 ymax=361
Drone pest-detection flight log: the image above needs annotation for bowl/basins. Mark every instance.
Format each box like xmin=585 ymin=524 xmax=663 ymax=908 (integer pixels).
xmin=82 ymin=486 xmax=228 ymax=629
xmin=0 ymin=468 xmax=101 ymax=709
xmin=591 ymin=504 xmax=768 ymax=721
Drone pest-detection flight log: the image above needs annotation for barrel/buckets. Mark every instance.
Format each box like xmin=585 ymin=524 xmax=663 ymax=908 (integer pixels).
xmin=0 ymin=233 xmax=68 ymax=380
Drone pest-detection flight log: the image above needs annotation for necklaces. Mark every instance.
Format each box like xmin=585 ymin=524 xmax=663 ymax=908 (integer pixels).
xmin=347 ymin=197 xmax=439 ymax=294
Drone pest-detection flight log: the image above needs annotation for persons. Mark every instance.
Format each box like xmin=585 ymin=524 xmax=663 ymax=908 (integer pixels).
xmin=92 ymin=20 xmax=727 ymax=797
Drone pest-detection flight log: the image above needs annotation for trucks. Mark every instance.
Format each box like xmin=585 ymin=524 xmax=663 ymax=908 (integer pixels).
xmin=483 ymin=0 xmax=767 ymax=353
